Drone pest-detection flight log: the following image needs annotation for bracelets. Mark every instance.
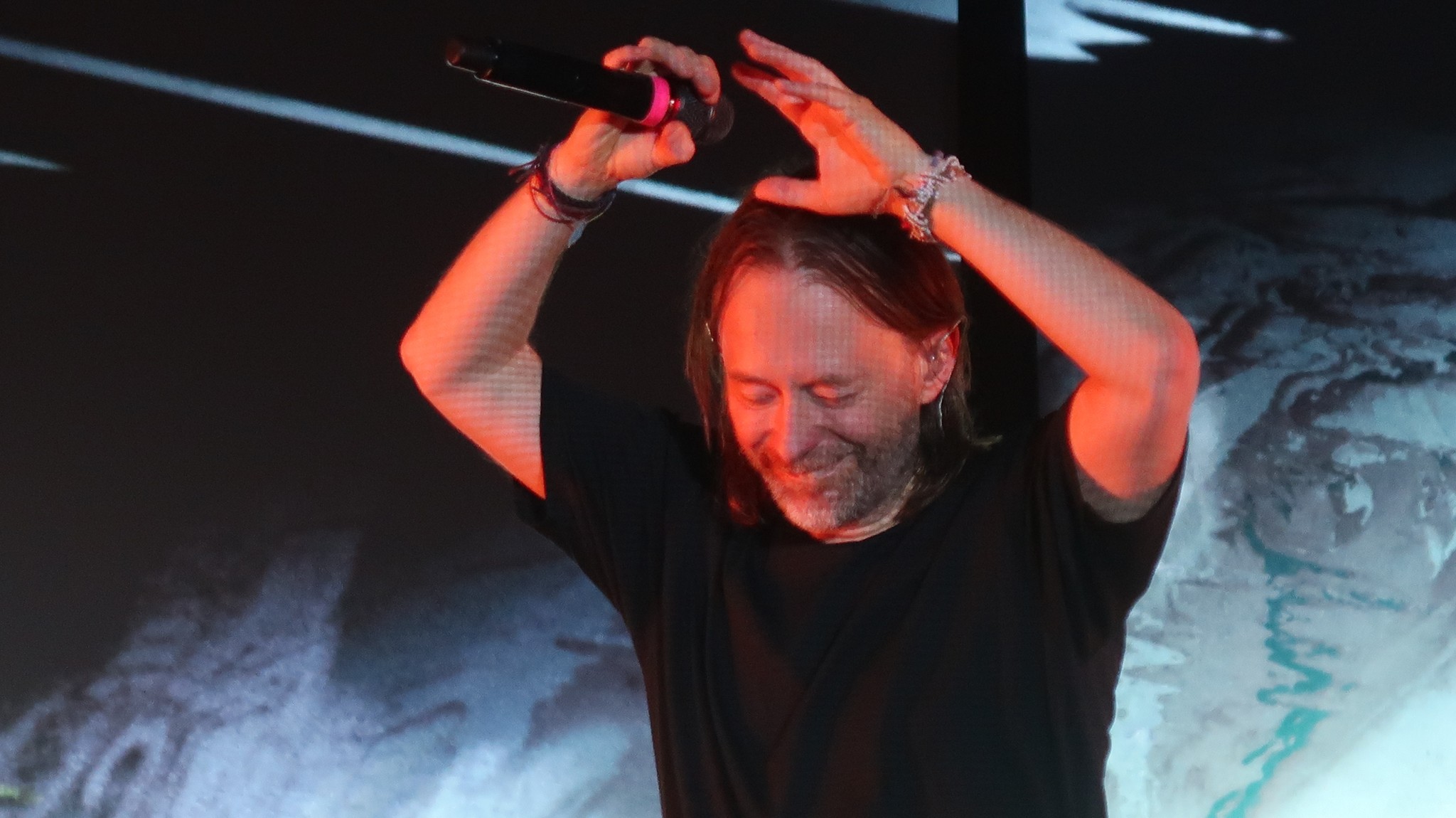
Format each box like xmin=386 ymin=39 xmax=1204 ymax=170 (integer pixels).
xmin=896 ymin=145 xmax=973 ymax=244
xmin=503 ymin=141 xmax=622 ymax=250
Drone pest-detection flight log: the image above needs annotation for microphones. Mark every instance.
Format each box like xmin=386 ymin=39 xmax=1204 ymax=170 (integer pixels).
xmin=443 ymin=42 xmax=736 ymax=143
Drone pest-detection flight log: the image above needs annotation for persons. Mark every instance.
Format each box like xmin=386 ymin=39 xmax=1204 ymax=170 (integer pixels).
xmin=398 ymin=5 xmax=1205 ymax=818
